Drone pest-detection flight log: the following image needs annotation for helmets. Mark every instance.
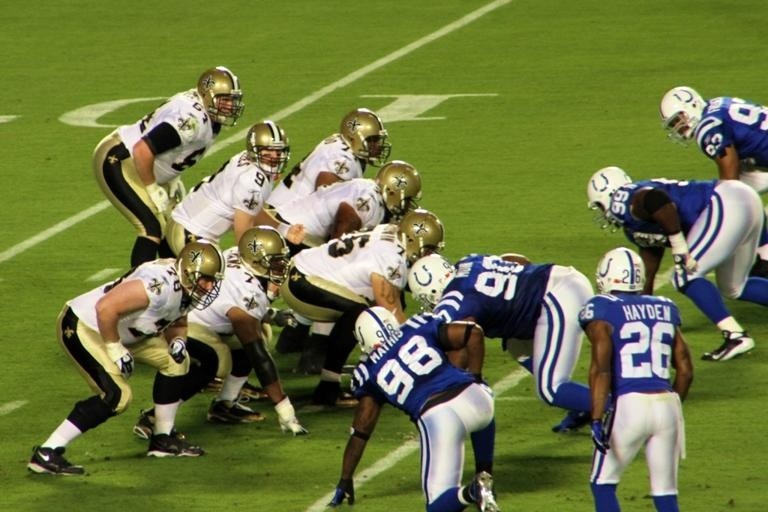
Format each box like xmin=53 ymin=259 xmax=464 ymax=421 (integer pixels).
xmin=246 ymin=123 xmax=291 ymax=182
xmin=377 ymin=160 xmax=459 ymax=310
xmin=355 ymin=304 xmax=402 ymax=351
xmin=342 ymin=109 xmax=391 ymax=169
xmin=599 ymin=246 xmax=646 ymax=296
xmin=175 ymin=244 xmax=226 ymax=311
xmin=198 ymin=66 xmax=244 ymax=128
xmin=237 ymin=226 xmax=292 ymax=286
xmin=587 ymin=165 xmax=635 ymax=233
xmin=661 ymin=86 xmax=710 ymax=150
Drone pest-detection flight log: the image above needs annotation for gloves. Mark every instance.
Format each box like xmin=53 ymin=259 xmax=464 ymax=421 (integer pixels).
xmin=269 ymin=308 xmax=297 ymax=328
xmin=168 ymin=338 xmax=186 ymax=364
xmin=675 ymin=253 xmax=699 ymax=277
xmin=170 ymin=175 xmax=186 ymax=202
xmin=274 ymin=396 xmax=309 ymax=435
xmin=325 ymin=477 xmax=355 ymax=508
xmin=147 ymin=181 xmax=171 ymax=214
xmin=108 ymin=339 xmax=136 ymax=381
xmin=591 ymin=419 xmax=611 ymax=456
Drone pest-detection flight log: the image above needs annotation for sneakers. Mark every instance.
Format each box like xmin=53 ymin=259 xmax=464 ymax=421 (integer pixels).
xmin=315 ymin=389 xmax=358 ymax=407
xmin=700 ymin=329 xmax=754 ymax=361
xmin=553 ymin=412 xmax=587 ymax=434
xmin=205 ymin=398 xmax=263 ymax=423
xmin=203 ymin=375 xmax=269 ymax=401
xmin=133 ymin=410 xmax=184 ymax=440
xmin=27 ymin=444 xmax=86 ymax=478
xmin=472 ymin=471 xmax=500 ymax=512
xmin=308 ymin=362 xmax=356 ymax=375
xmin=147 ymin=428 xmax=204 ymax=459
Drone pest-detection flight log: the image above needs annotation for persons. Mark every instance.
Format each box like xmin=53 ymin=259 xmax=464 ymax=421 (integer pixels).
xmin=578 ymin=247 xmax=693 ymax=512
xmin=660 ymin=85 xmax=768 ymax=278
xmin=132 ymin=107 xmax=446 ymax=440
xmin=328 ymin=252 xmax=610 ymax=512
xmin=587 ymin=166 xmax=768 ymax=362
xmin=95 ymin=66 xmax=244 ymax=271
xmin=28 ymin=240 xmax=225 ymax=474
